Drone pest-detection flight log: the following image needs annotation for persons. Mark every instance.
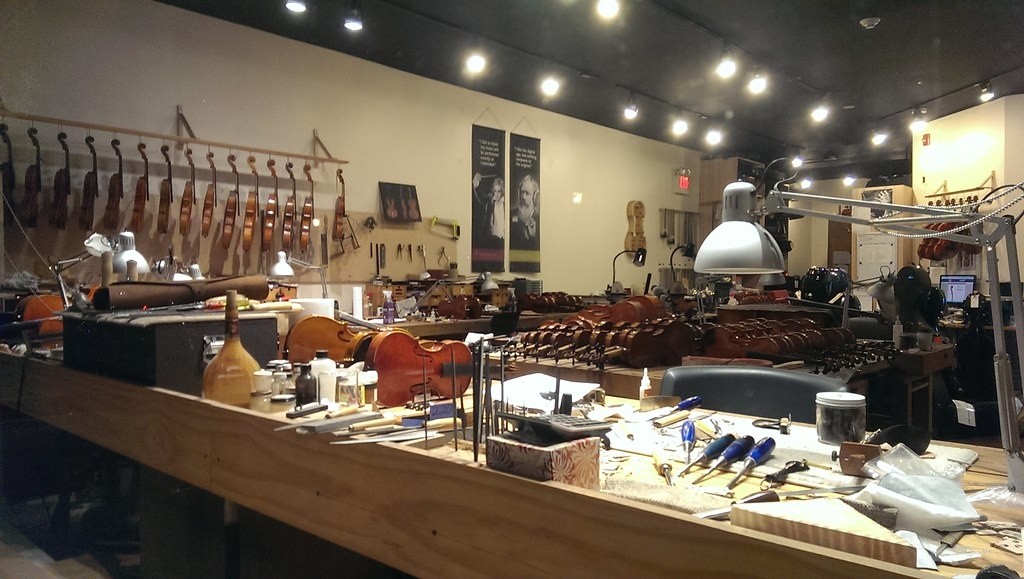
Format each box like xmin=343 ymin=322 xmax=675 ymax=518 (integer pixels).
xmin=472 ymin=171 xmax=540 ymax=247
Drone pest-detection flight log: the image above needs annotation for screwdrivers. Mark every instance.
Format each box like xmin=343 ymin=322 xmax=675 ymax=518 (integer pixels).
xmin=645 ymin=396 xmax=703 ymax=423
xmin=648 ymin=448 xmax=675 ymax=487
xmin=678 ymin=419 xmax=776 ymax=496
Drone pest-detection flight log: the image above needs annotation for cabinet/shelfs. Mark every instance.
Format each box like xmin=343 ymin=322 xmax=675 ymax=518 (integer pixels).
xmin=698 ymin=158 xmax=790 ymax=268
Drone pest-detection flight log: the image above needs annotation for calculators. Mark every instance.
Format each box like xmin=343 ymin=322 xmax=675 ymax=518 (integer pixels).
xmin=497 ymin=406 xmax=612 ymax=443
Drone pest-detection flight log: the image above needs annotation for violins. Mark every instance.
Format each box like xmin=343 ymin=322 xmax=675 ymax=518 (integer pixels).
xmin=201 ymin=152 xmax=218 ymax=239
xmin=435 ymin=294 xmax=482 ymax=319
xmin=366 ymin=329 xmax=521 ymax=410
xmin=332 ymin=170 xmax=346 ymax=242
xmin=300 ymin=165 xmax=316 ymax=253
xmin=260 ymin=160 xmax=278 ymax=252
xmin=281 ymin=162 xmax=298 ymax=249
xmin=282 ymin=318 xmax=409 ymax=366
xmin=492 ymin=292 xmax=904 ymax=375
xmin=179 ymin=149 xmax=197 ymax=237
xmin=343 ymin=329 xmax=442 ymax=402
xmin=242 ymin=156 xmax=260 ymax=252
xmin=15 ymin=283 xmax=101 ymax=339
xmin=157 ymin=144 xmax=174 ymax=234
xmin=0 ymin=124 xmax=149 ymax=235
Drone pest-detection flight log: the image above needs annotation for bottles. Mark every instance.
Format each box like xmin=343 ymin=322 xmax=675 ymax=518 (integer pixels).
xmin=202 ymin=290 xmax=260 ymax=409
xmin=266 ymin=364 xmax=276 ymax=374
xmin=430 ymin=308 xmax=435 ymax=322
xmin=274 ymin=372 xmax=286 ymax=395
xmin=309 ymin=350 xmax=337 ymax=404
xmin=293 ymin=362 xmax=302 ymax=380
xmin=382 ymin=290 xmax=395 ymax=324
xmin=282 ymin=363 xmax=293 ymax=379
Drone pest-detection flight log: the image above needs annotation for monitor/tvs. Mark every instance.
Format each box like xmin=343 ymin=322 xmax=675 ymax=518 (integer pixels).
xmin=940 ymin=274 xmax=976 ymax=307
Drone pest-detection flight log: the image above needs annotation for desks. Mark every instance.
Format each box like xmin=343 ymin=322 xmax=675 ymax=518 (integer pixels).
xmin=482 ymin=330 xmax=818 ymax=408
xmin=856 ymin=339 xmax=954 ymax=440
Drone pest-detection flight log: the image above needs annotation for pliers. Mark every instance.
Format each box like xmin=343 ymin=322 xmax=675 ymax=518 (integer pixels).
xmin=438 ymin=246 xmax=450 ymax=265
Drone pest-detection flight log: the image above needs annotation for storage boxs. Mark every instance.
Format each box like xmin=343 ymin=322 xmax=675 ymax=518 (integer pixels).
xmin=484 ymin=429 xmax=602 ymax=496
xmin=62 ymin=310 xmax=279 ymax=399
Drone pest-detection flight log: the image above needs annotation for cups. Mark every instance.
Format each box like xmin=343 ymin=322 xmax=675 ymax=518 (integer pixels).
xmin=296 ymin=364 xmax=317 ymax=406
xmin=338 ymin=369 xmax=359 ymax=409
xmin=254 ymin=368 xmax=272 ymax=392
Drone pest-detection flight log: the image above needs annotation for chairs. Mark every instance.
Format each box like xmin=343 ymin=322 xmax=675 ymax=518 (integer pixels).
xmin=893 ymin=266 xmax=942 ymax=336
xmin=661 ymin=366 xmax=847 ymax=434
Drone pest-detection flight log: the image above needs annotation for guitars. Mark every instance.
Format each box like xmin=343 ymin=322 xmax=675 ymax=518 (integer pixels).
xmin=221 ymin=154 xmax=240 ymax=250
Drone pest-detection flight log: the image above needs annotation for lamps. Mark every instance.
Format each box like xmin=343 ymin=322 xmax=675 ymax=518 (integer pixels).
xmin=605 ymin=247 xmax=647 ymax=297
xmin=694 ymin=181 xmax=1024 ymax=495
xmin=677 ymin=167 xmax=692 ymax=189
